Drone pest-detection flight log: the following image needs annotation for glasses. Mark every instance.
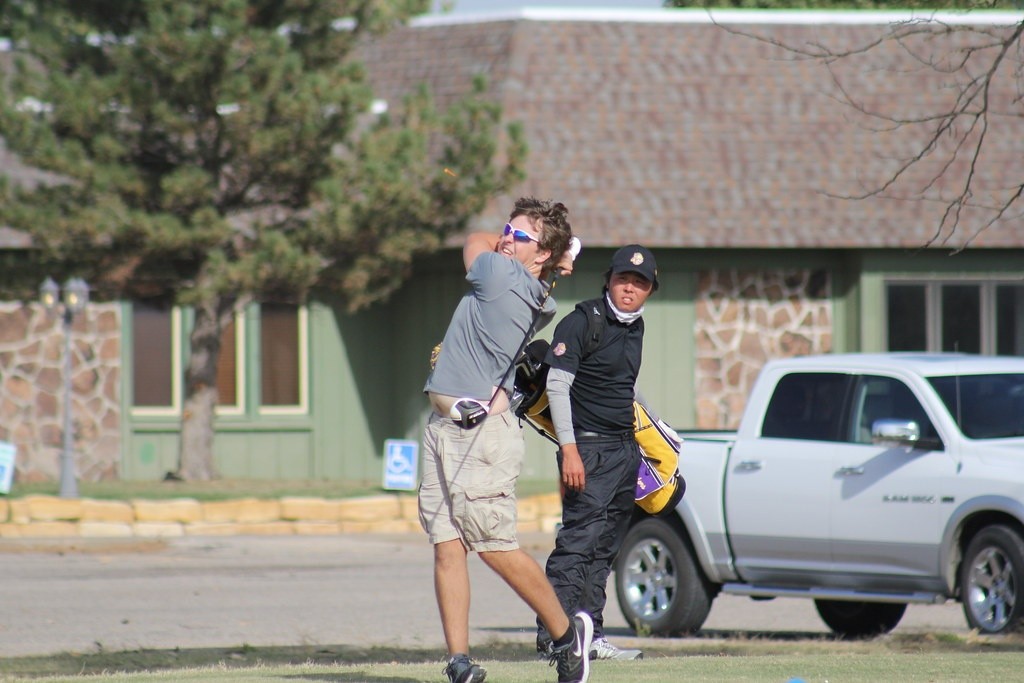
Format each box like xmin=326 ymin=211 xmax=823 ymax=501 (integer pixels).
xmin=504 ymin=223 xmax=540 ymax=243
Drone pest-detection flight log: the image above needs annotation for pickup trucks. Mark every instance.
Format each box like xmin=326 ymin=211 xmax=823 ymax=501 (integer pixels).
xmin=555 ymin=349 xmax=1023 ymax=640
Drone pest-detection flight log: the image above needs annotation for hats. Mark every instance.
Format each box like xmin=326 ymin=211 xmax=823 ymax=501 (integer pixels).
xmin=613 ymin=244 xmax=657 ymax=282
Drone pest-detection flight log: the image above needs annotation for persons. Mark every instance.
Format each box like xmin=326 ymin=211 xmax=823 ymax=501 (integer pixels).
xmin=415 ymin=196 xmax=595 ymax=683
xmin=535 ymin=243 xmax=660 ymax=660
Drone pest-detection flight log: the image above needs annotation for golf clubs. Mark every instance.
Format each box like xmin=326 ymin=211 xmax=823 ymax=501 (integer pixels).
xmin=449 ymin=235 xmax=582 ymax=431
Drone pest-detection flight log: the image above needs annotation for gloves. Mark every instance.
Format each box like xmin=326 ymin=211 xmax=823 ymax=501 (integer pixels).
xmin=568 ymin=235 xmax=582 ymax=259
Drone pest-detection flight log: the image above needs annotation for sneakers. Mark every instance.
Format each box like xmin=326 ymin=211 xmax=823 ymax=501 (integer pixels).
xmin=547 ymin=612 xmax=593 ymax=683
xmin=442 ymin=656 xmax=487 ymax=683
xmin=537 ymin=632 xmax=597 ymax=660
xmin=590 ymin=637 xmax=643 ymax=660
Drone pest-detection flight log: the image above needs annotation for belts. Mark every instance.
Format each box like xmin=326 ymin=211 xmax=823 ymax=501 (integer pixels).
xmin=575 ymin=431 xmax=632 ymax=439
